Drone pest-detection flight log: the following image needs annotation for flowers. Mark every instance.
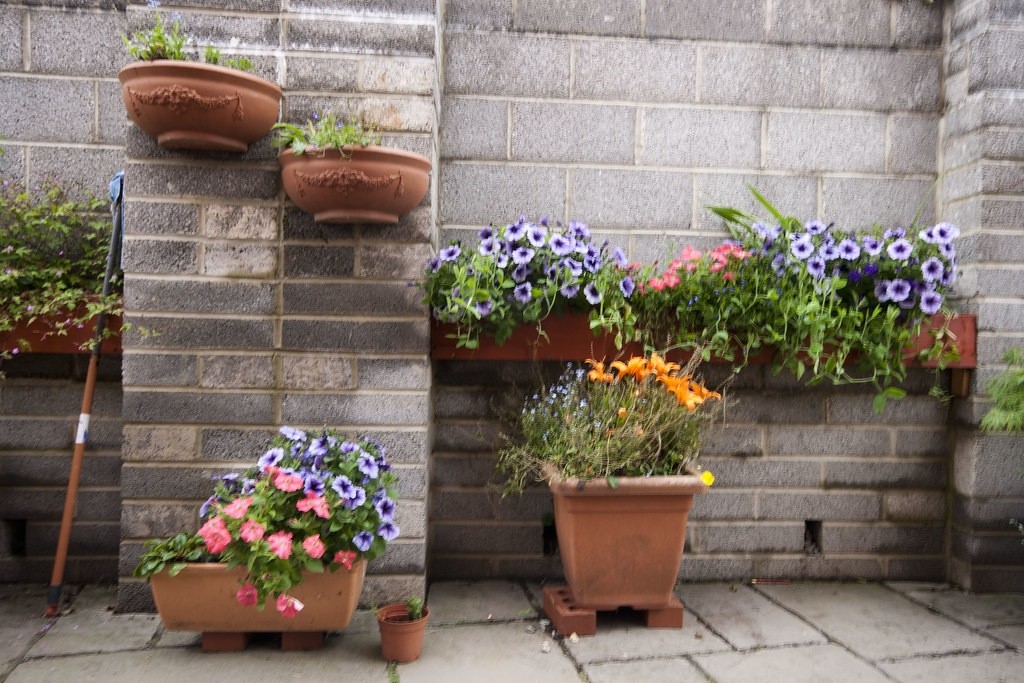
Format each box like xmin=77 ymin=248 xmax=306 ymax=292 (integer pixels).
xmin=0 ymin=179 xmax=124 ymax=358
xmin=133 ymin=426 xmax=399 ymax=619
xmin=268 ymin=109 xmax=381 ymax=157
xmin=417 ymin=213 xmax=955 ymax=408
xmin=121 ymin=1 xmax=257 ymax=71
xmin=492 ymin=352 xmax=720 ymax=486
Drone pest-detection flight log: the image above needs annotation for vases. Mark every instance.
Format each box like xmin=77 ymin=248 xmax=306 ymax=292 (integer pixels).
xmin=149 ymin=555 xmax=368 ymax=632
xmin=118 ymin=59 xmax=283 ymax=154
xmin=427 ymin=297 xmax=979 ymax=373
xmin=277 ymin=145 xmax=433 ymax=225
xmin=540 ymin=453 xmax=708 ymax=611
xmin=0 ymin=300 xmax=124 ymax=358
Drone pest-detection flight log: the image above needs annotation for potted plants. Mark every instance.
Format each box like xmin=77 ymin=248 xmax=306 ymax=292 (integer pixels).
xmin=377 ymin=596 xmax=430 ymax=662
